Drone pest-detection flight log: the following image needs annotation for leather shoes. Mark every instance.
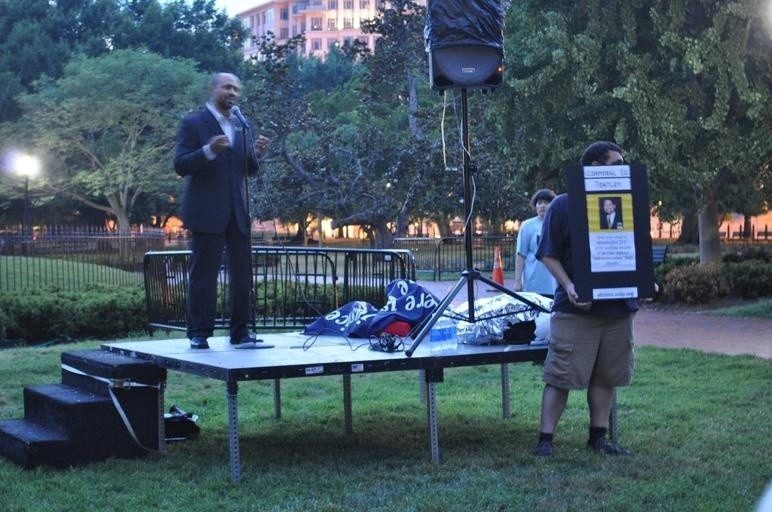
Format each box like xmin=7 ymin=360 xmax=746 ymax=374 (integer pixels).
xmin=229 ymin=336 xmax=254 ymax=344
xmin=191 ymin=335 xmax=208 ymax=349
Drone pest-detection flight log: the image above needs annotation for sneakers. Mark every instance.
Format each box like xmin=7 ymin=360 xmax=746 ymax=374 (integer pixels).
xmin=585 ymin=437 xmax=634 ymax=459
xmin=534 ymin=440 xmax=553 ymax=455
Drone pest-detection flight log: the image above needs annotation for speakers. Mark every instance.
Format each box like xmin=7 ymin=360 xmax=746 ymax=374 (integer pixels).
xmin=424 ymin=1 xmax=507 ymax=91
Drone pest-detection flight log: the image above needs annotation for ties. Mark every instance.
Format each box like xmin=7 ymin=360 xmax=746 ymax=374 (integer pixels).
xmin=607 ymin=216 xmax=612 ymax=229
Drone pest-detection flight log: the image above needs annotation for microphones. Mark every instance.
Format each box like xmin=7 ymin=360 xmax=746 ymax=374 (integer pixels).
xmin=230 ymin=105 xmax=250 ymax=130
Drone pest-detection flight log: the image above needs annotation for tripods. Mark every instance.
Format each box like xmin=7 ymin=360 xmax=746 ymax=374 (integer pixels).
xmin=405 ymin=92 xmax=554 ymax=360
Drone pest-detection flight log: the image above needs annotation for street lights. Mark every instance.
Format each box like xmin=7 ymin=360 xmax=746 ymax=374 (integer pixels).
xmin=12 ymin=152 xmax=45 ymax=234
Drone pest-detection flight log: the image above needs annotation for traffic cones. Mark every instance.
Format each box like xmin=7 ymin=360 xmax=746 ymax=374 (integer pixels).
xmin=484 ymin=249 xmax=507 ymax=292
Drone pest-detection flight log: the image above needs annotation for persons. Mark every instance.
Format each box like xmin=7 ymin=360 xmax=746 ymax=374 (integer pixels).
xmin=600 ymin=197 xmax=622 ymax=230
xmin=513 ymin=187 xmax=560 ymax=300
xmin=532 ymin=140 xmax=635 ymax=458
xmin=174 ymin=72 xmax=265 ymax=349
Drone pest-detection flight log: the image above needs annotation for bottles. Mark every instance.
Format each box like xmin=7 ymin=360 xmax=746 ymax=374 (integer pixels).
xmin=429 ymin=314 xmax=441 ymax=353
xmin=441 ymin=313 xmax=450 ymax=350
xmin=450 ymin=313 xmax=457 ymax=349
